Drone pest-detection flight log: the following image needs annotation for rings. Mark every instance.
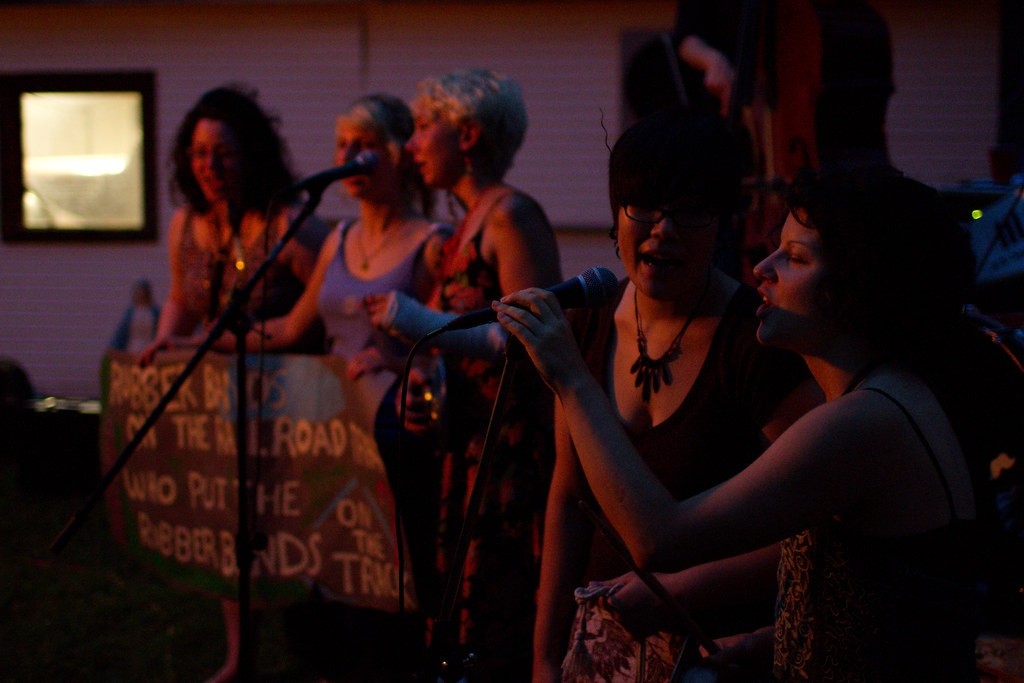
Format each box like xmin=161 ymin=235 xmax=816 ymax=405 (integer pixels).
xmin=370 ymin=293 xmax=376 ymax=303
xmin=362 ymin=295 xmax=366 ymax=306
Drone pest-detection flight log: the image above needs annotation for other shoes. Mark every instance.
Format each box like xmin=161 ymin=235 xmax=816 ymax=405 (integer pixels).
xmin=462 ymin=650 xmax=486 ymax=676
xmin=202 ymin=658 xmax=257 ymax=683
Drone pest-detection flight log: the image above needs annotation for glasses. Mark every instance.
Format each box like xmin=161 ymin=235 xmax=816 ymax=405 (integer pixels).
xmin=618 ymin=197 xmax=723 ymax=228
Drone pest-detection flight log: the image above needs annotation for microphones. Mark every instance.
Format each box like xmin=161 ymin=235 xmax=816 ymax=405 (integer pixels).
xmin=442 ymin=265 xmax=619 ymax=331
xmin=284 ymin=150 xmax=381 ymax=198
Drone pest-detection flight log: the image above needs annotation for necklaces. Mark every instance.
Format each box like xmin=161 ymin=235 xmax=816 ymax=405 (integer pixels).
xmin=357 ymin=221 xmax=405 ymax=269
xmin=630 ymin=284 xmax=699 ymax=403
xmin=203 ymin=209 xmax=233 ymax=322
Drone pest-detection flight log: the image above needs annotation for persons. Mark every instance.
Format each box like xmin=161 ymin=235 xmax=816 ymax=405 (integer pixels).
xmin=138 ymin=0 xmax=1024 ymax=683
